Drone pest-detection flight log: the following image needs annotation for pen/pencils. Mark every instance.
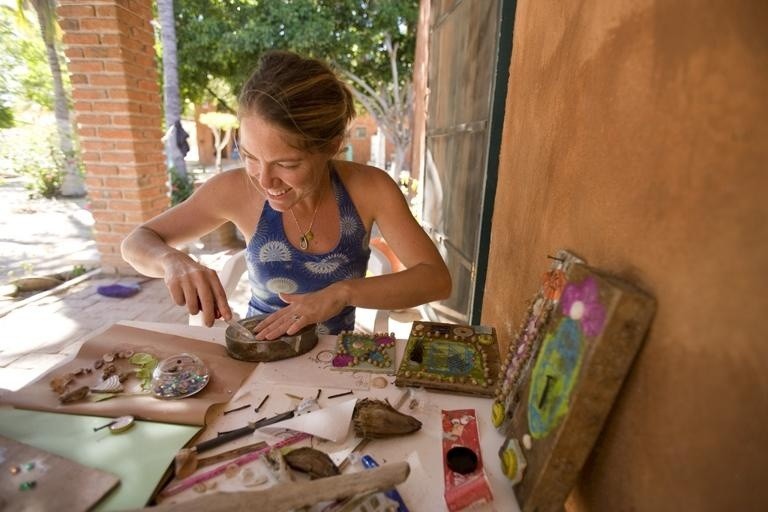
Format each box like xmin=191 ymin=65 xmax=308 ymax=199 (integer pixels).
xmin=362 ymin=455 xmax=409 ymax=512
xmin=159 ymin=432 xmax=314 ymax=498
xmin=190 ymin=411 xmax=293 ymax=454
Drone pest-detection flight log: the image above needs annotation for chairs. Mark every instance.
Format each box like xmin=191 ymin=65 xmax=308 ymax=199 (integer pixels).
xmin=189 ymin=239 xmax=400 ymax=340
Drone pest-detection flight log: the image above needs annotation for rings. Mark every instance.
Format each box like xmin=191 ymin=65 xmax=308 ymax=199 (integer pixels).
xmin=291 ymin=314 xmax=302 ymax=322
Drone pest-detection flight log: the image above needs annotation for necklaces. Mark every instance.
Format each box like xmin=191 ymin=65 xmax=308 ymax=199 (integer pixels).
xmin=287 ymin=177 xmax=326 ymax=251
xmin=493 ymin=254 xmax=567 ymax=404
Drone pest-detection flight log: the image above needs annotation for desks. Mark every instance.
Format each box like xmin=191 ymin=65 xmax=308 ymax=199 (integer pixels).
xmin=2 ymin=317 xmax=521 ymax=512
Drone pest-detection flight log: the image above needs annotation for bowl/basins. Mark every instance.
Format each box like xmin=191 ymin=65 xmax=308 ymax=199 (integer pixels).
xmin=224 ymin=313 xmax=318 ymax=361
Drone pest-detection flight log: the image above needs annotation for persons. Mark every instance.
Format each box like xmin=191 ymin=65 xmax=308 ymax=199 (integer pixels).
xmin=121 ymin=49 xmax=452 ymax=340
